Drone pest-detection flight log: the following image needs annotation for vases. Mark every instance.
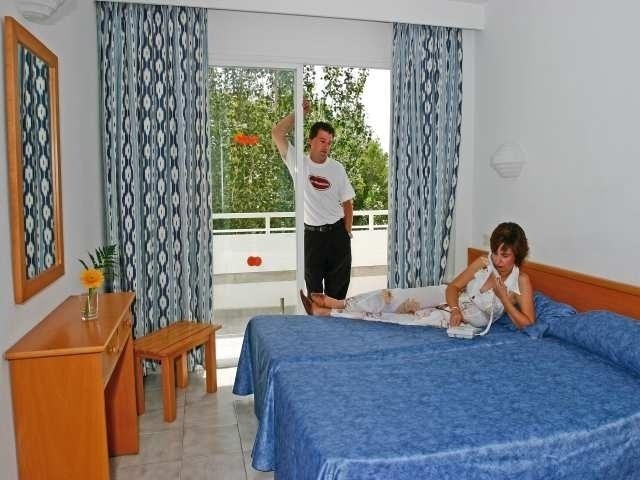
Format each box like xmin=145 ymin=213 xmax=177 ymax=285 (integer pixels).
xmin=81 ymin=295 xmax=98 ymax=321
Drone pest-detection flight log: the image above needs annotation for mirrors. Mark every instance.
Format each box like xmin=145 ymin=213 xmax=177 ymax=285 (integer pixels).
xmin=4 ymin=16 xmax=66 ymax=305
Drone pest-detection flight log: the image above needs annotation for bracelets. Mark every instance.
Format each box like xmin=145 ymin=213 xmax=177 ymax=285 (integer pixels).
xmin=449 ymin=307 xmax=459 ymax=313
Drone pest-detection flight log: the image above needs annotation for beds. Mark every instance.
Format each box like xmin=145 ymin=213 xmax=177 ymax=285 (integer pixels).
xmin=232 ymin=243 xmax=639 ymax=480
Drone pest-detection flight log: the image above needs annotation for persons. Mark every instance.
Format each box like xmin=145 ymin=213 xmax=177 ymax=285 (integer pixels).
xmin=271 ymin=100 xmax=356 ymax=300
xmin=301 ymin=223 xmax=536 ymax=329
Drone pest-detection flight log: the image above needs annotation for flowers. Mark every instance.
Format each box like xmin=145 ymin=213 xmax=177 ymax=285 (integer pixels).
xmin=78 ymin=245 xmax=122 ymax=317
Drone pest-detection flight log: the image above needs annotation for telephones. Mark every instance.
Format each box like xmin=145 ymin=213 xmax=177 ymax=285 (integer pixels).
xmin=487 ymin=251 xmax=502 ymax=278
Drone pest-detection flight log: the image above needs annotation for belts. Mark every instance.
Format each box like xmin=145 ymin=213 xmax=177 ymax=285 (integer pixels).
xmin=304 ymin=224 xmax=329 ymax=232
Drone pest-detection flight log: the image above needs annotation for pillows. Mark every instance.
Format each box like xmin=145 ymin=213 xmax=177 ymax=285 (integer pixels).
xmin=546 ymin=310 xmax=640 ymax=375
xmin=494 ymin=291 xmax=578 ymax=339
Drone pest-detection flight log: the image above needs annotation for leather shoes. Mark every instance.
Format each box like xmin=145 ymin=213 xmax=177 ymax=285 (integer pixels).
xmin=310 ymin=293 xmax=332 ymax=309
xmin=300 ymin=290 xmax=312 ymax=315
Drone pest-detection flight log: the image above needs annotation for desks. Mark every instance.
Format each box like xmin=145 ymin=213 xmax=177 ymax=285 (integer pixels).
xmin=3 ymin=291 xmax=140 ymax=479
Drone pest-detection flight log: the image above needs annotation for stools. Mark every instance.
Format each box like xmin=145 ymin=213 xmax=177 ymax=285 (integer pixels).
xmin=134 ymin=321 xmax=221 ymax=423
